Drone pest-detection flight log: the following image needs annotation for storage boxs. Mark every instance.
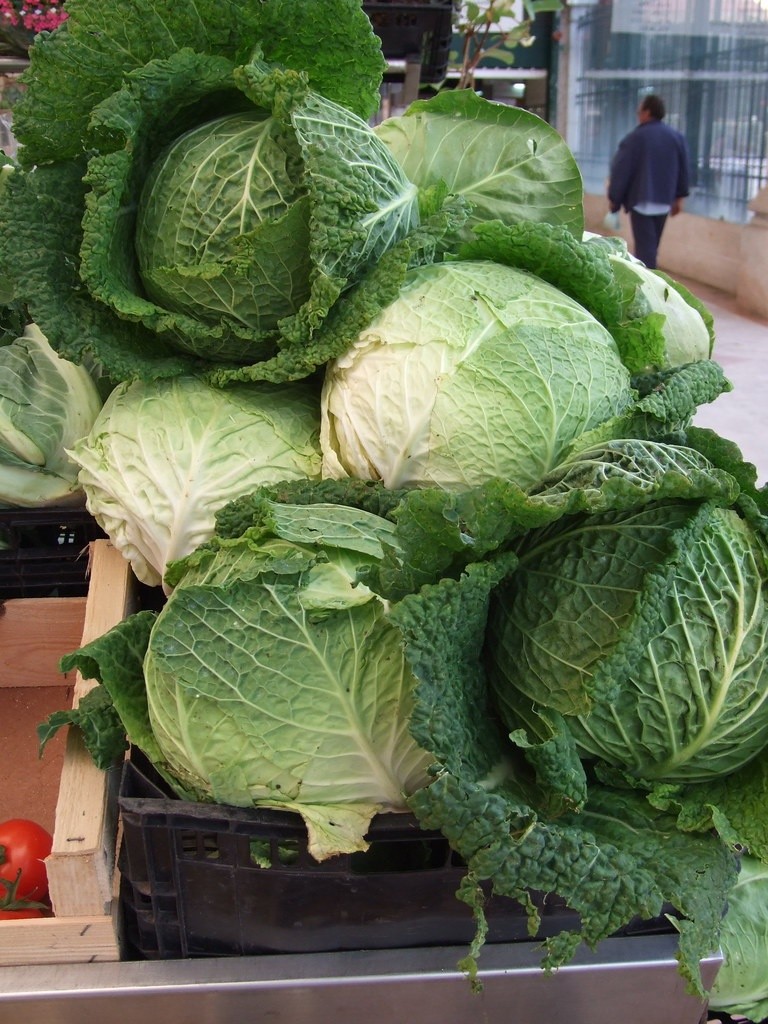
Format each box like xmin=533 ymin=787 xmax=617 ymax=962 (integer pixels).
xmin=0 ymin=509 xmax=167 ymax=608
xmin=0 ymin=538 xmax=140 ymax=967
xmin=117 ymin=755 xmax=690 ymax=962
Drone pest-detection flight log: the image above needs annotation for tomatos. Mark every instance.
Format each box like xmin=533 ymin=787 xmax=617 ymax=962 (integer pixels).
xmin=0 ymin=867 xmax=51 ymax=920
xmin=0 ymin=819 xmax=54 ymax=903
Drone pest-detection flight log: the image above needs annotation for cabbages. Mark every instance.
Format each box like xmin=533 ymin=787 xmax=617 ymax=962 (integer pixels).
xmin=0 ymin=0 xmax=768 ymax=1024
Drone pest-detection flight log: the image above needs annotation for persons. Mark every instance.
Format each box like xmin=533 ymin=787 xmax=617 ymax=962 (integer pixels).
xmin=606 ymin=93 xmax=688 ymax=272
